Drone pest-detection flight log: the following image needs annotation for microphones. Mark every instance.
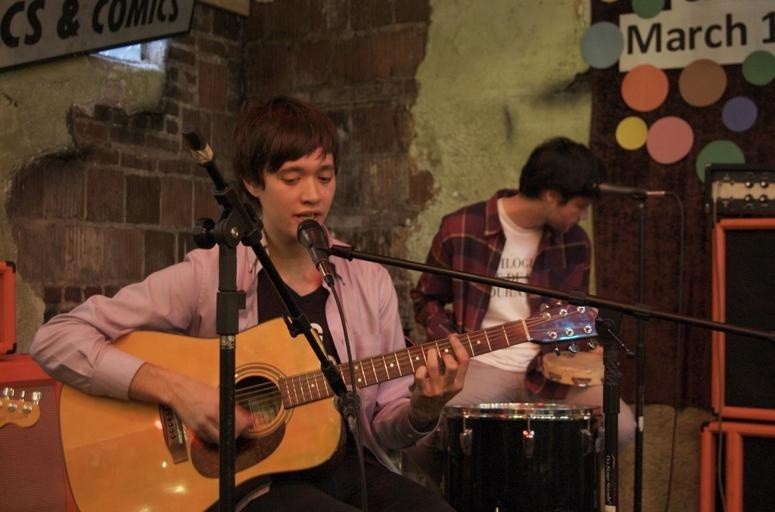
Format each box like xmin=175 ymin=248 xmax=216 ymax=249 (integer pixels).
xmin=296 ymin=218 xmax=337 ymax=289
xmin=583 ymin=179 xmax=674 ymax=201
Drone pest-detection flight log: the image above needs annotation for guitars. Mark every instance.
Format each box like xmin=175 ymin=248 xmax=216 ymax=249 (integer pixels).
xmin=58 ymin=303 xmax=599 ymax=512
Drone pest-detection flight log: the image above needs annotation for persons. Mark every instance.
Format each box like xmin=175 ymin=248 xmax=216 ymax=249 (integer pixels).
xmin=31 ymin=95 xmax=470 ymax=512
xmin=410 ymin=136 xmax=637 ymax=468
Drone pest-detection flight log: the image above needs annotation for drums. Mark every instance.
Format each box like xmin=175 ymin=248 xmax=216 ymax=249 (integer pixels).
xmin=443 ymin=400 xmax=601 ymax=512
xmin=543 ymin=344 xmax=605 ymax=387
